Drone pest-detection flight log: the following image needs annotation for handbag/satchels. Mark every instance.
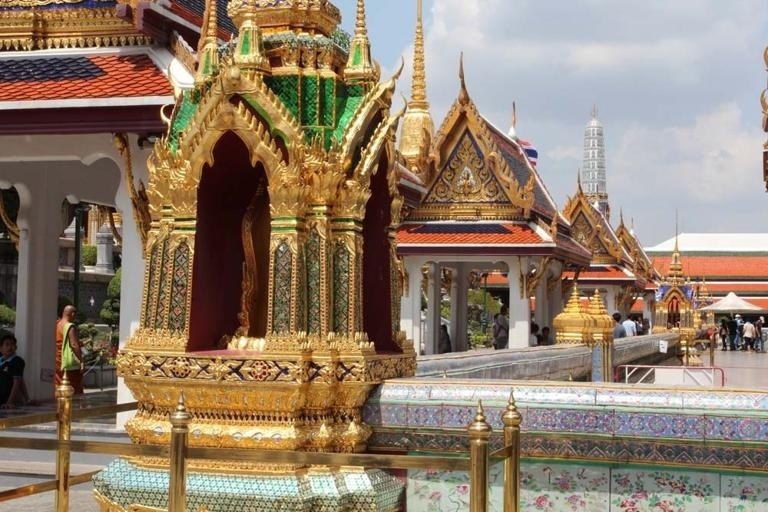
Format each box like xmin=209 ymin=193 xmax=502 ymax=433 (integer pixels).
xmin=61 ymin=322 xmax=81 ymax=371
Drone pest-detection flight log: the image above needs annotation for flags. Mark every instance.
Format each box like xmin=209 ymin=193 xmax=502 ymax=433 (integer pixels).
xmin=516 ymin=138 xmax=537 ymax=170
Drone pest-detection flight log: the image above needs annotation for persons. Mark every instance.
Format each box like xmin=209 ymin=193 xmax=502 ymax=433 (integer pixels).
xmin=612 ymin=312 xmax=626 ymax=338
xmin=635 ymin=317 xmax=643 ymax=327
xmin=440 ymin=324 xmax=451 ymax=353
xmin=493 ymin=305 xmax=555 ymax=350
xmin=719 ymin=314 xmax=765 ymax=352
xmin=0 ymin=333 xmax=25 ymax=409
xmin=10 ymin=335 xmax=40 ymax=406
xmin=56 ymin=305 xmax=83 ymax=394
xmin=623 ymin=313 xmax=637 ymax=337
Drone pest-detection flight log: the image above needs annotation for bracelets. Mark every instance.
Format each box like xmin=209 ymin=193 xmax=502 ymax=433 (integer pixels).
xmin=25 ymin=399 xmax=32 ymax=403
